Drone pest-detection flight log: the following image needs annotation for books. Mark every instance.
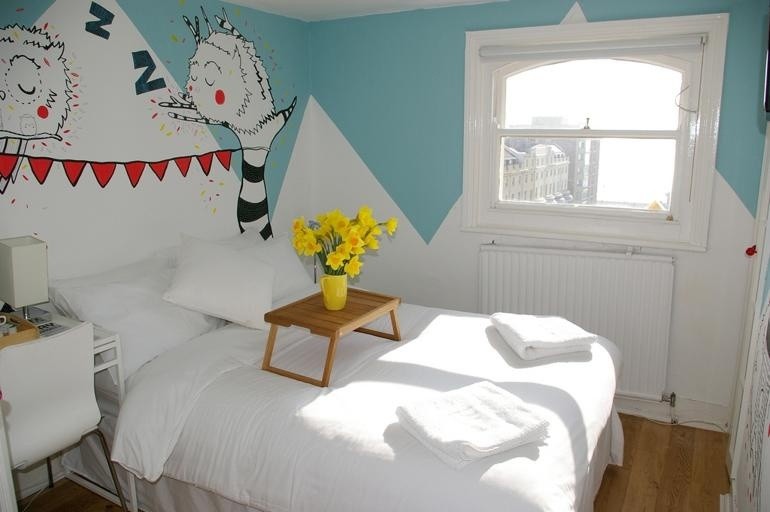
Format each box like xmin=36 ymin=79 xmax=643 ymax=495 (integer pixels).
xmin=30 ymin=317 xmax=69 ymax=338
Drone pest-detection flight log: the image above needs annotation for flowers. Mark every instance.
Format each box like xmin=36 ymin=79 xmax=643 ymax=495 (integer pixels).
xmin=289 ymin=204 xmax=399 ymax=274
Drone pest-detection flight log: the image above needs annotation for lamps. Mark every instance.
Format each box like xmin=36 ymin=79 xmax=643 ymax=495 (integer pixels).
xmin=0 ymin=235 xmax=49 ymax=327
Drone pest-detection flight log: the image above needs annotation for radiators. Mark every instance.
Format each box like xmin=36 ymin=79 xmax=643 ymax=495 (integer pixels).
xmin=474 ymin=242 xmax=679 ymax=406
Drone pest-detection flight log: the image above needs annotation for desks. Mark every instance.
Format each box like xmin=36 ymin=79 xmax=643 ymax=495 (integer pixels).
xmin=261 ymin=285 xmax=403 ymax=387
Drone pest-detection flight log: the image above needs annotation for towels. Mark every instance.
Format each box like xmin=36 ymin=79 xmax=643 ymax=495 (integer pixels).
xmin=489 ymin=310 xmax=600 ymax=363
xmin=392 ymin=377 xmax=551 ymax=475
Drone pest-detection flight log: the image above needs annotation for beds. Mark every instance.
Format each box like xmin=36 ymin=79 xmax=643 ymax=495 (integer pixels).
xmin=60 ymin=281 xmax=624 ymax=512
